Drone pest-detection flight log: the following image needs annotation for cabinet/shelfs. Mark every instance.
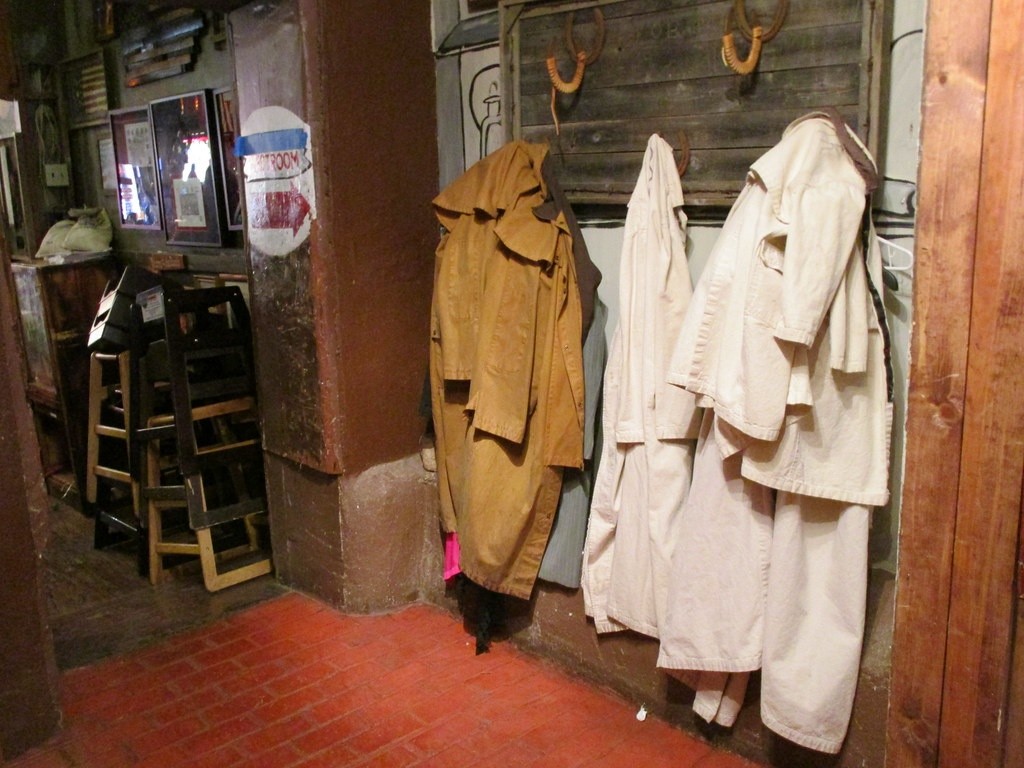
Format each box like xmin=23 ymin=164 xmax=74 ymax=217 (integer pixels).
xmin=9 ymin=246 xmax=124 ymax=511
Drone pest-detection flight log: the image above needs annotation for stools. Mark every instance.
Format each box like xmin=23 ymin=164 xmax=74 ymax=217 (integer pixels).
xmin=86 ymin=265 xmax=272 ymax=595
